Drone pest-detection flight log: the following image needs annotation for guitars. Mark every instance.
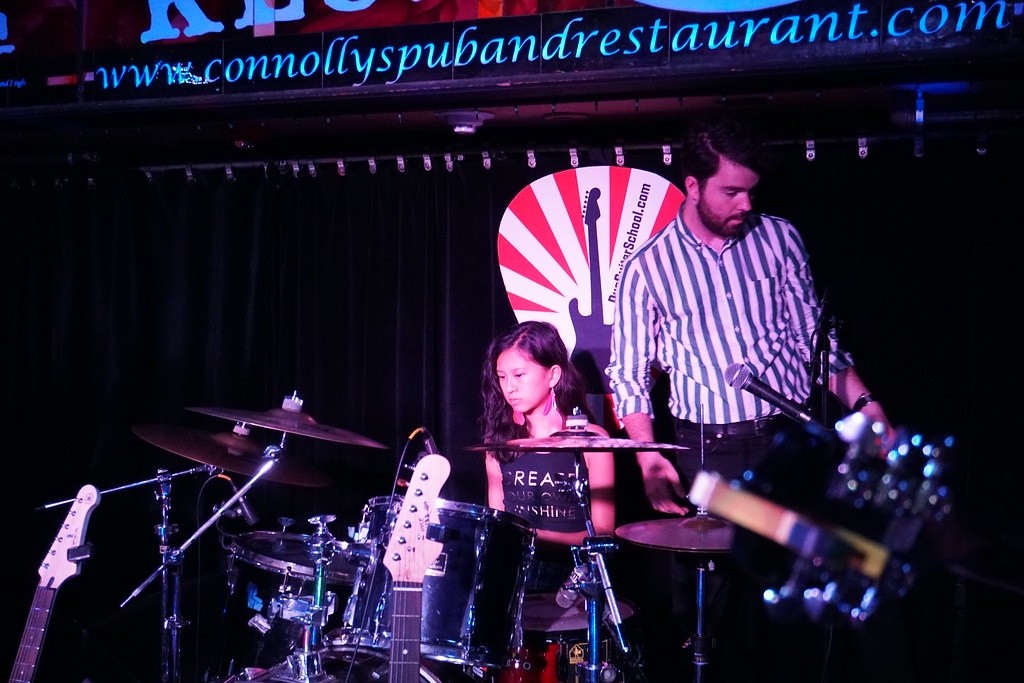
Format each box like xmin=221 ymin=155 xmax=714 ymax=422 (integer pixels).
xmin=6 ymin=483 xmax=104 ymax=683
xmin=381 ymin=448 xmax=452 ymax=683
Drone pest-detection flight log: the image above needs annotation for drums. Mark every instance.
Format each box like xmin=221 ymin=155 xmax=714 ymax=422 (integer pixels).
xmin=222 ymin=491 xmax=638 ymax=683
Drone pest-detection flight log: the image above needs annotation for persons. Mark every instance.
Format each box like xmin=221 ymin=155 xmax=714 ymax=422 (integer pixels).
xmin=604 ymin=116 xmax=897 ymax=516
xmin=476 ymin=322 xmax=616 ymax=595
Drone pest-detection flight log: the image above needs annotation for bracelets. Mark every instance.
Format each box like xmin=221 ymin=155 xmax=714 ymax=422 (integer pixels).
xmin=853 ymin=393 xmax=878 ymax=412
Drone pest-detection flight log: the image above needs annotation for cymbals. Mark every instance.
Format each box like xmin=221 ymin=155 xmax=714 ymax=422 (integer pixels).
xmin=614 ymin=517 xmax=735 ymax=554
xmin=130 ymin=423 xmax=335 ymax=488
xmin=459 ymin=427 xmax=692 ymax=452
xmin=185 ymin=405 xmax=389 ymax=450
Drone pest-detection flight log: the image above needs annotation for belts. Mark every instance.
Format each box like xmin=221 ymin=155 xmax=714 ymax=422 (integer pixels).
xmin=678 ymin=413 xmax=793 ymax=437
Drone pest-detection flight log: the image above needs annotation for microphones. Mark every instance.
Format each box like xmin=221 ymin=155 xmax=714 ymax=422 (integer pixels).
xmin=420 ymin=426 xmax=439 ymax=456
xmin=724 ymin=363 xmax=819 ymax=427
xmin=556 ymin=564 xmax=589 ymax=608
xmin=231 ymin=484 xmax=258 ymax=526
